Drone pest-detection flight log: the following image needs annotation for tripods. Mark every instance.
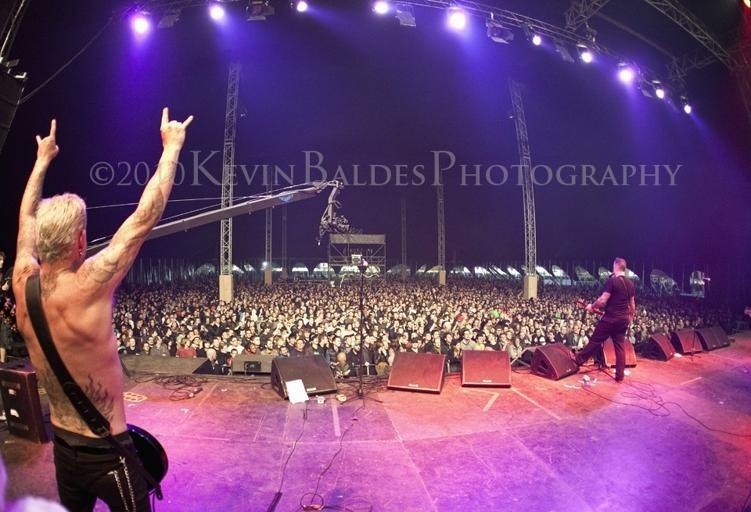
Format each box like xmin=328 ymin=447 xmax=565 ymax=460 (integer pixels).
xmin=578 ymin=338 xmax=618 ymax=380
xmin=339 ymin=307 xmax=385 ymax=411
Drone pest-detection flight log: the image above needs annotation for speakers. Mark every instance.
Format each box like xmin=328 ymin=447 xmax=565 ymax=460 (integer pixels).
xmin=695 ymin=325 xmax=720 ymax=350
xmin=598 ymin=339 xmax=639 ymax=370
xmin=671 ymin=328 xmax=704 ymax=355
xmin=387 ymin=350 xmax=448 ymax=395
xmin=270 ymin=353 xmax=340 ymax=402
xmin=458 ymin=347 xmax=514 ymax=392
xmin=641 ymin=335 xmax=676 ymax=362
xmin=531 ymin=342 xmax=581 ymax=382
xmin=710 ymin=324 xmax=730 ymax=348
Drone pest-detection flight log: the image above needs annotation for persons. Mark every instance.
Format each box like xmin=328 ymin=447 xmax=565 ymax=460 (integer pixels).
xmin=11 ymin=108 xmax=195 ymax=512
xmin=113 ymin=292 xmax=232 ymax=377
xmin=575 ymin=258 xmax=636 ymax=381
xmin=635 ymin=282 xmax=738 ymax=343
xmin=0 ymin=251 xmax=10 ymax=294
xmin=1 ymin=294 xmax=20 ymax=360
xmin=129 ymin=273 xmax=219 ymax=292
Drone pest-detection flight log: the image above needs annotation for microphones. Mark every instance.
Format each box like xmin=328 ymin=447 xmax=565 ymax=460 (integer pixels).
xmin=605 ymin=271 xmax=616 ymax=279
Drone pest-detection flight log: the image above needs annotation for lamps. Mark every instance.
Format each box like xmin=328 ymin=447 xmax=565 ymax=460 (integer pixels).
xmin=244 ymin=1 xmax=276 ymax=22
xmin=484 ymin=19 xmax=514 ymax=45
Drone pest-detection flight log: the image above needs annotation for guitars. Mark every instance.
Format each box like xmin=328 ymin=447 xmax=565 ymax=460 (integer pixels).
xmin=0 ymin=294 xmax=168 ymax=492
xmin=576 ymin=298 xmax=604 ymax=317
